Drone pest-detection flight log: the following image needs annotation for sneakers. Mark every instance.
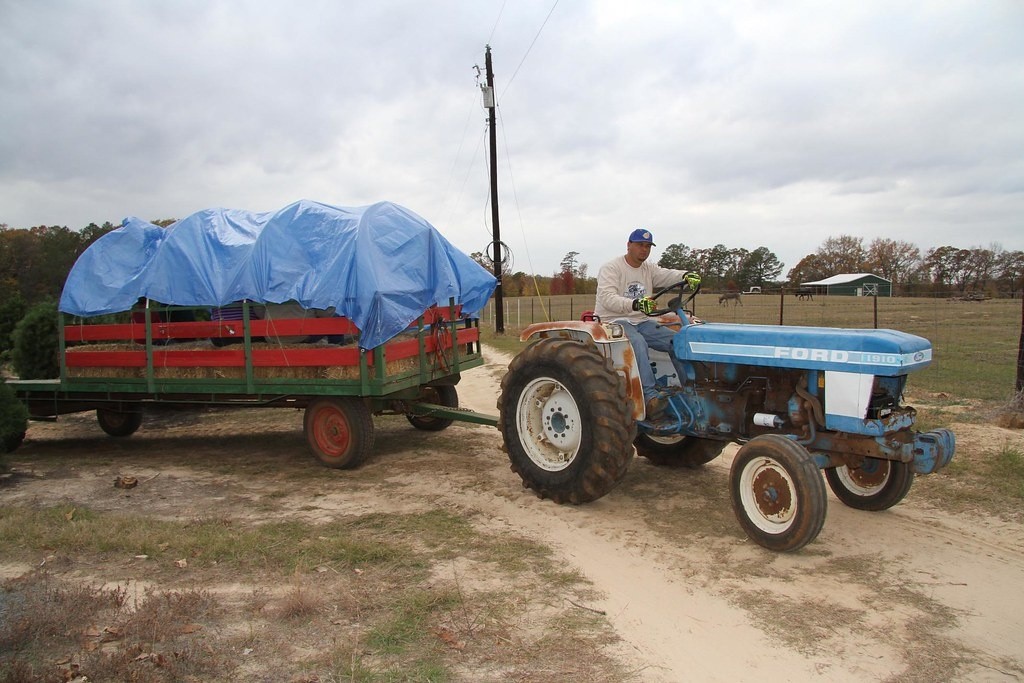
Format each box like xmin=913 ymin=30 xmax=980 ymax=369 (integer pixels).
xmin=648 ymin=391 xmax=667 ymax=415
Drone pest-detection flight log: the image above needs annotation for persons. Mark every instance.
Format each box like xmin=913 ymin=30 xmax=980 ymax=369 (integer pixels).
xmin=252 ymin=297 xmax=344 ymax=347
xmin=592 ymin=229 xmax=702 ymax=424
xmin=129 ymin=295 xmax=163 ymax=346
xmin=160 ymin=300 xmax=200 ymax=345
xmin=208 ymin=299 xmax=267 ymax=346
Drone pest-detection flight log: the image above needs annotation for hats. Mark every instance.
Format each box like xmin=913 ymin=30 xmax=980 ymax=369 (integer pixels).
xmin=629 ymin=229 xmax=656 ymax=246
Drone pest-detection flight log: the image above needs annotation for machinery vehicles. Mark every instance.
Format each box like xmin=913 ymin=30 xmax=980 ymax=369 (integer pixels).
xmin=1 ymin=201 xmax=954 ymax=552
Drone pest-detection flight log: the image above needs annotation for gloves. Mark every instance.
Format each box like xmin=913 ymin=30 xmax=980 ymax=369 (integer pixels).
xmin=684 ymin=273 xmax=702 ymax=289
xmin=632 ymin=296 xmax=656 ymax=314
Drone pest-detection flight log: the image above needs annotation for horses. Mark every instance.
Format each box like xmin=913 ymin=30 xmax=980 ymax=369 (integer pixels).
xmin=718 ymin=293 xmax=743 ymax=308
xmin=795 ymin=290 xmax=814 ymax=301
